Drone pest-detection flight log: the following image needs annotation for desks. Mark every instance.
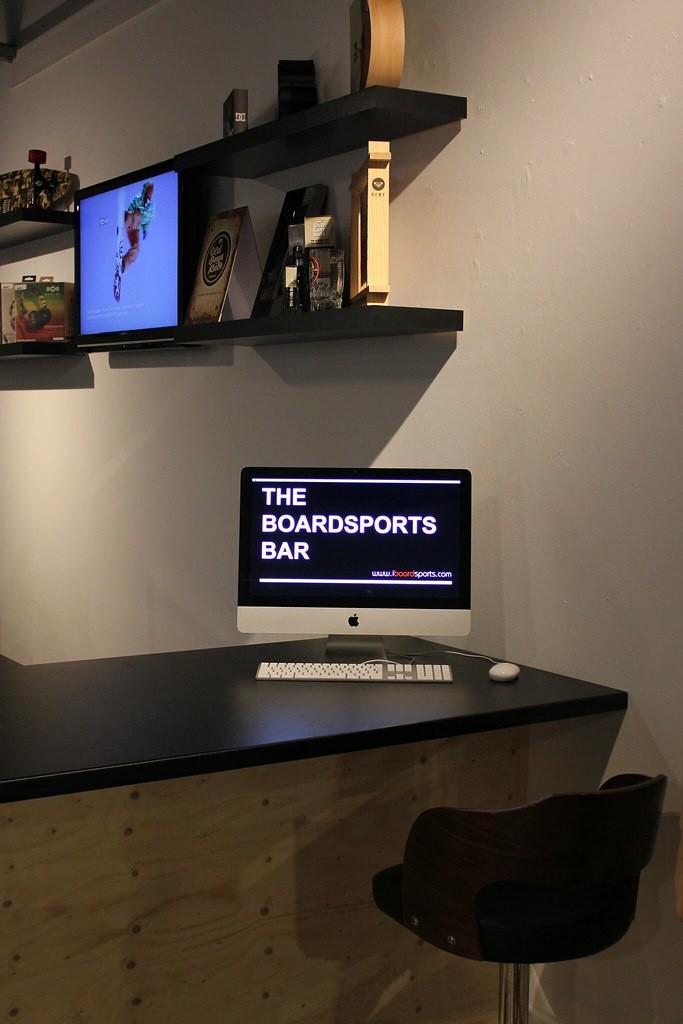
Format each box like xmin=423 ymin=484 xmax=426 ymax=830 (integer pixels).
xmin=0 ymin=635 xmax=629 ymax=1023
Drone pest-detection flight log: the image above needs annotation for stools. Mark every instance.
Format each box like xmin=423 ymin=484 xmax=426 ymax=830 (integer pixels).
xmin=374 ymin=772 xmax=668 ymax=1024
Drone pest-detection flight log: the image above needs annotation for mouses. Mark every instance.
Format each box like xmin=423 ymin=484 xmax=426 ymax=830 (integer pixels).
xmin=489 ymin=662 xmax=520 ymax=682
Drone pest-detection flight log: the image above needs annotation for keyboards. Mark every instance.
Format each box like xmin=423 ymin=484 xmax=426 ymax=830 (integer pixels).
xmin=254 ymin=662 xmax=453 ymax=683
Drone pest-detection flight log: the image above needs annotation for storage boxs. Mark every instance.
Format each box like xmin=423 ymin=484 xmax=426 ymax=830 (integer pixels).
xmin=303 ymin=216 xmax=335 ymax=248
xmin=13 ymin=282 xmax=74 ymax=342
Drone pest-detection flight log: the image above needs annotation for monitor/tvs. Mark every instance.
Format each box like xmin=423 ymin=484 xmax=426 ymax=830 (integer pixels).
xmin=73 ymin=159 xmax=197 ymax=351
xmin=236 ymin=466 xmax=472 ymax=663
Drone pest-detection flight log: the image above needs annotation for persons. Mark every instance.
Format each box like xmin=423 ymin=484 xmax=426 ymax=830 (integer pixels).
xmin=120 ymin=180 xmax=156 ymax=273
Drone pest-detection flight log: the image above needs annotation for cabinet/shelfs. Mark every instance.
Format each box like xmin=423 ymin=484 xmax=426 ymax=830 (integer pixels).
xmin=0 ymin=85 xmax=467 ymax=360
